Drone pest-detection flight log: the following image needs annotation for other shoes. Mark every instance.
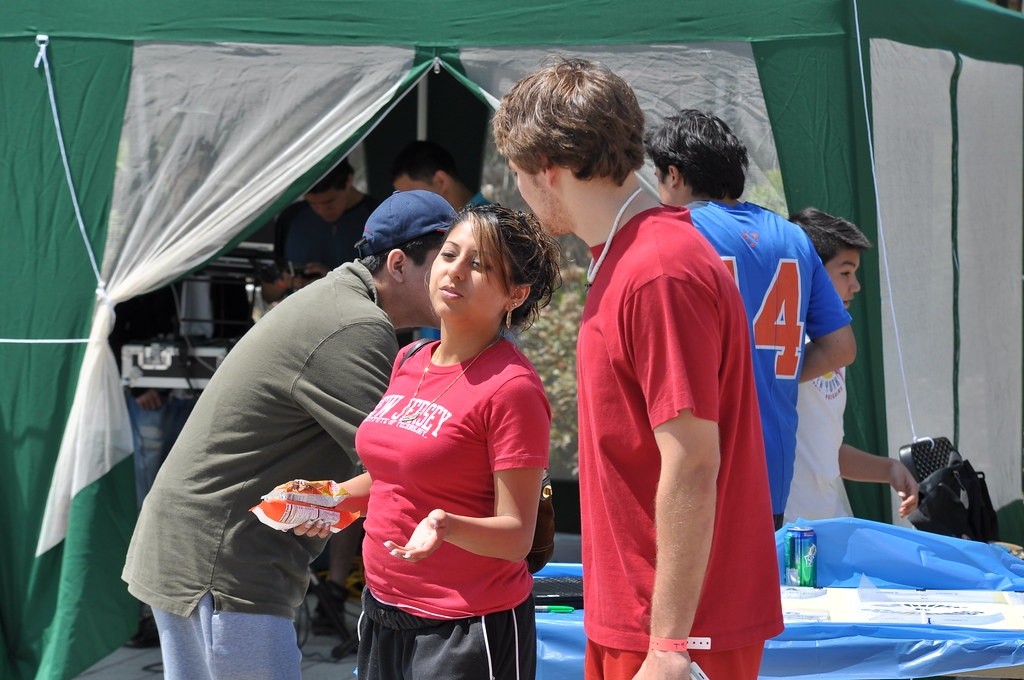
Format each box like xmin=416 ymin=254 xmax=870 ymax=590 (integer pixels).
xmin=313 ymin=580 xmax=350 ymax=636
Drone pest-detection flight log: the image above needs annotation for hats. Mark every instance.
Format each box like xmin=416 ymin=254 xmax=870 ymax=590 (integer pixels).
xmin=354 ymin=189 xmax=458 ymax=259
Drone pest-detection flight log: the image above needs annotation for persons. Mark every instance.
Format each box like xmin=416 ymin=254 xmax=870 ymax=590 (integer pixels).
xmin=292 ymin=198 xmax=554 ymax=680
xmin=492 ymin=60 xmax=786 ymax=680
xmin=119 ymin=190 xmax=460 ymax=680
xmin=256 ymin=139 xmax=522 ymax=349
xmin=641 ymin=107 xmax=920 ymax=533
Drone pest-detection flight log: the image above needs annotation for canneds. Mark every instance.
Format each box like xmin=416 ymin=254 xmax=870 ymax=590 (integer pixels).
xmin=785 ymin=526 xmax=817 ymax=588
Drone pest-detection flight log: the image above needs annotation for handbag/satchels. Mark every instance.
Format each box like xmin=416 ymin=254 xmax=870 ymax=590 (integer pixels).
xmin=525 ymin=472 xmax=555 ymax=572
xmin=908 ymin=451 xmax=1000 ymax=542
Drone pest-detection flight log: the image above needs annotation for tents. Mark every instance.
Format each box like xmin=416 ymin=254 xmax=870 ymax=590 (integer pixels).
xmin=0 ymin=0 xmax=1024 ymax=679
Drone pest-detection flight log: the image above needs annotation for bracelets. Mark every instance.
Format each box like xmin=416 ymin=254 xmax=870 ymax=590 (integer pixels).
xmin=649 ymin=636 xmax=712 ymax=651
